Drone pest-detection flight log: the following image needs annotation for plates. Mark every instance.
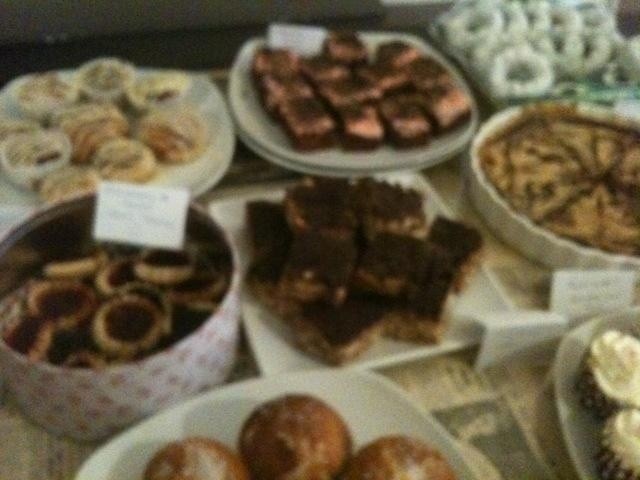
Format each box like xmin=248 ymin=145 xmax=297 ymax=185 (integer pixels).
xmin=4 ymin=70 xmax=232 ymax=200
xmin=225 ymin=34 xmax=478 ymax=179
xmin=5 ymin=191 xmax=241 ymax=444
xmin=465 ymin=104 xmax=640 ymax=285
xmin=212 ymin=172 xmax=514 ymax=382
xmin=428 ymin=18 xmax=639 ymax=107
xmin=74 ymin=369 xmax=479 ymax=479
xmin=554 ymin=310 xmax=640 ymax=476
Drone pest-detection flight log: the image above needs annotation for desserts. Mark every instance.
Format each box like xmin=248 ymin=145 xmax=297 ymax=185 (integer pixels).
xmin=0 ymin=0 xmax=640 ymax=480
